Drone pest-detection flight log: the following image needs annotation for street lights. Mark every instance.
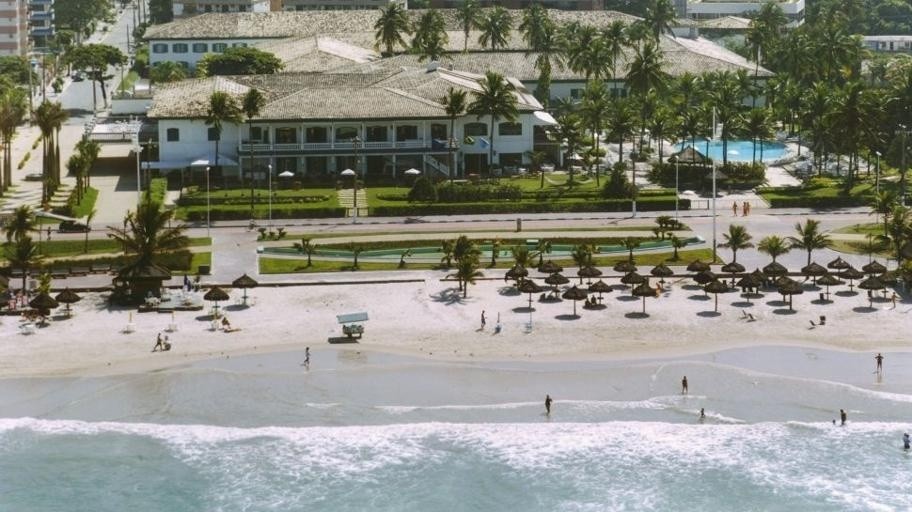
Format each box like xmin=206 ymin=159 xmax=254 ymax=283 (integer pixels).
xmin=873 ymin=150 xmax=882 ymax=202
xmin=206 ymin=166 xmax=211 ymax=236
xmin=673 ymin=154 xmax=680 ymax=224
xmin=710 ymin=105 xmax=727 ymax=264
xmin=266 ymin=164 xmax=272 ymax=224
xmin=897 ymin=123 xmax=905 ymax=208
xmin=129 ymin=146 xmax=142 ymax=203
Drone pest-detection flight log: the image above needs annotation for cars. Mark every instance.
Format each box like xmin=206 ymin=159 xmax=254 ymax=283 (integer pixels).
xmin=71 ymin=70 xmax=86 ymax=80
xmin=59 ymin=219 xmax=91 ymax=234
xmin=0 ymin=217 xmax=9 ymax=229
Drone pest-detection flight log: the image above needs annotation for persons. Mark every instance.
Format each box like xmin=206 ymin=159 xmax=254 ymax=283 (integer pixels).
xmin=742 ymin=202 xmax=747 ymax=216
xmin=303 ymin=346 xmax=312 ymax=364
xmin=544 ymin=394 xmax=553 ymax=416
xmin=700 ymin=407 xmax=704 ymax=419
xmin=874 ymin=352 xmax=884 ymax=371
xmin=733 ymin=201 xmax=738 ymax=217
xmin=891 ymin=292 xmax=899 ymax=307
xmin=746 ymin=202 xmax=751 ymax=215
xmin=222 ymin=316 xmax=232 ymax=330
xmin=903 ymin=433 xmax=911 ymax=448
xmin=480 ymin=309 xmax=486 ymax=328
xmin=839 ymin=408 xmax=848 ymax=425
xmin=681 ymin=375 xmax=689 ymax=395
xmin=154 ymin=332 xmax=163 ymax=352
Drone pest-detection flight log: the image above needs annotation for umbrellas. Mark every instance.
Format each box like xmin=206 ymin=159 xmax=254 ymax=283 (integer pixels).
xmin=30 ymin=290 xmax=59 ymax=317
xmin=204 ymin=286 xmax=230 ymax=318
xmin=55 ymin=286 xmax=81 ymax=313
xmin=233 ymin=272 xmax=258 ymax=305
